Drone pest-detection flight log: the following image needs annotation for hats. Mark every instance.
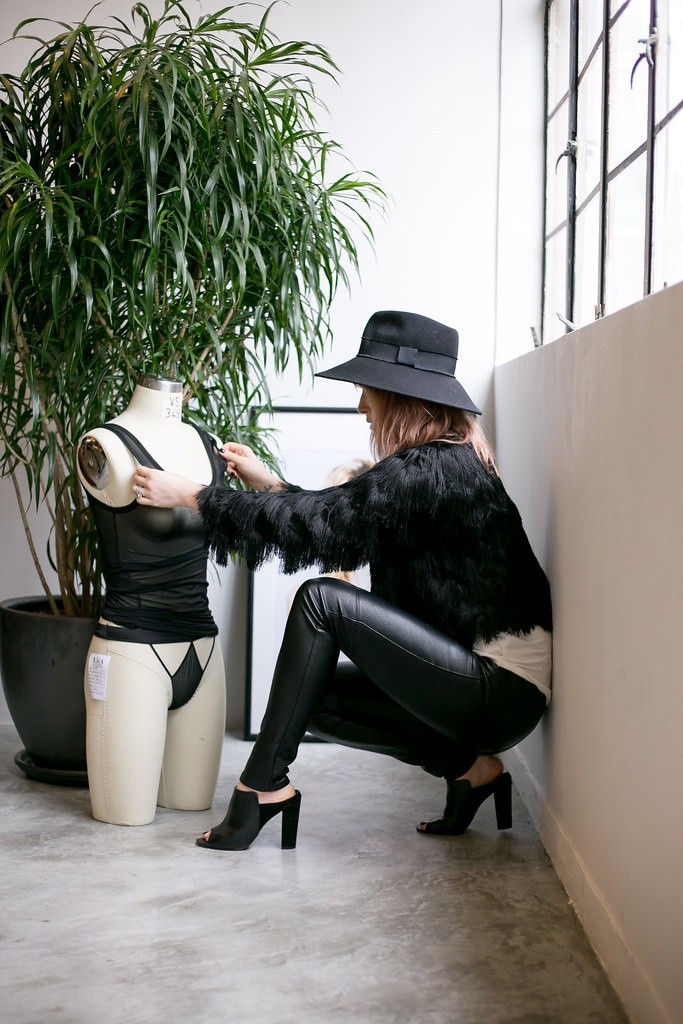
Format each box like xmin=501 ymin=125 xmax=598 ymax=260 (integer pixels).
xmin=315 ymin=312 xmax=482 ymax=415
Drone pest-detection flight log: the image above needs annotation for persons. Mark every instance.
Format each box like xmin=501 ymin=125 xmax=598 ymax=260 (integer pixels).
xmin=131 ymin=312 xmax=554 ymax=851
xmin=77 ymin=372 xmax=229 ymax=829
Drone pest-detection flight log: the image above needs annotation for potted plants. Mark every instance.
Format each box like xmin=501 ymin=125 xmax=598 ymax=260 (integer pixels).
xmin=0 ymin=0 xmax=388 ymax=788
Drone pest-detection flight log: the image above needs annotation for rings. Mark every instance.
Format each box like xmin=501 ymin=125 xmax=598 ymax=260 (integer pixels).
xmin=136 ymin=486 xmax=141 ymax=497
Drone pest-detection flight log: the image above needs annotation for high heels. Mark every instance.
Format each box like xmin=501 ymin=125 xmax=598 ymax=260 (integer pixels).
xmin=196 ymin=784 xmax=301 ymax=850
xmin=415 ymin=771 xmax=513 ymax=837
